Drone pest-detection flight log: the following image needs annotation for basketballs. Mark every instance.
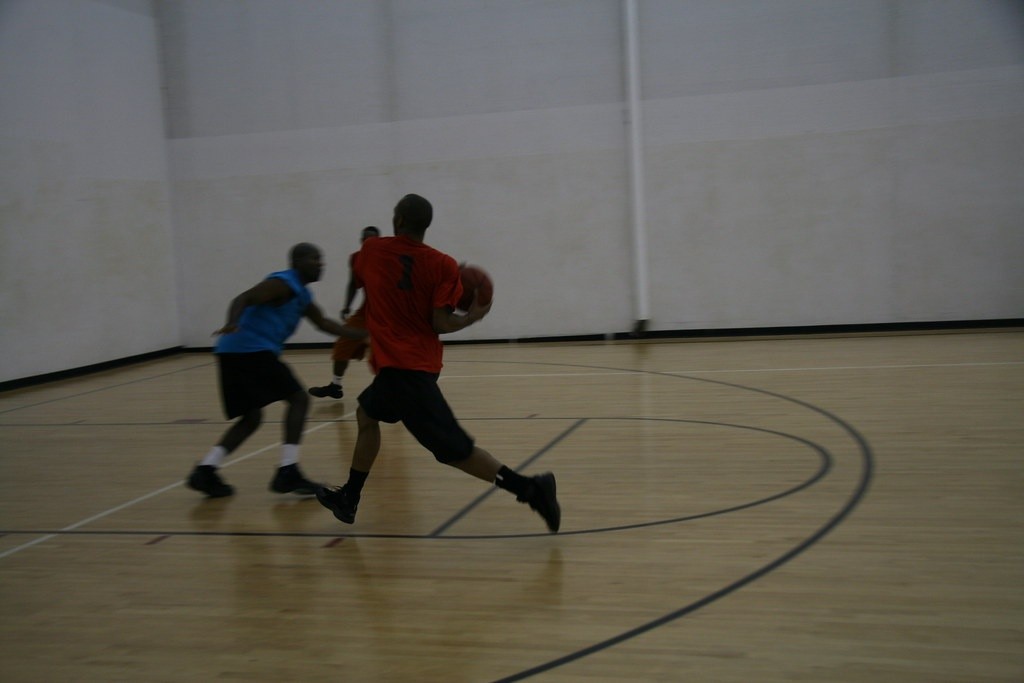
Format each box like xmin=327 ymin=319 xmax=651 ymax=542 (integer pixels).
xmin=455 ymin=265 xmax=494 ymax=312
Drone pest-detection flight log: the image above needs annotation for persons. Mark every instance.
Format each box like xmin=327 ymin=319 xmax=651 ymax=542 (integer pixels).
xmin=316 ymin=194 xmax=561 ymax=534
xmin=187 ymin=243 xmax=368 ymax=497
xmin=309 ymin=227 xmax=380 ymax=399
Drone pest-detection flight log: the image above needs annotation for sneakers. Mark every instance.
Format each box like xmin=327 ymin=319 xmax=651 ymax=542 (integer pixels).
xmin=269 ymin=463 xmax=326 ymax=496
xmin=315 ymin=483 xmax=359 ymax=524
xmin=515 ymin=472 xmax=560 ymax=535
xmin=308 ymin=381 xmax=343 ymax=399
xmin=185 ymin=463 xmax=238 ymax=497
